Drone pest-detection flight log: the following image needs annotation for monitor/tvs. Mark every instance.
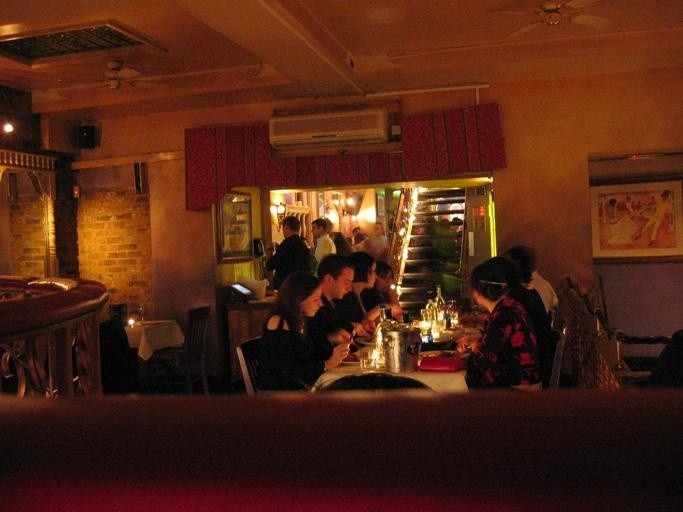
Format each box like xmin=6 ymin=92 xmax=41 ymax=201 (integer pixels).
xmin=225 ymin=282 xmax=256 ymax=303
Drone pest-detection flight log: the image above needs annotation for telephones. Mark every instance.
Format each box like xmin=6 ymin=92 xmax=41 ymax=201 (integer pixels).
xmin=254 ymin=238 xmax=263 ymax=257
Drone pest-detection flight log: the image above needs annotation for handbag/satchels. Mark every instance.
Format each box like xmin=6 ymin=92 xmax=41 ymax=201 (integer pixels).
xmin=420 ymin=356 xmax=465 ymax=372
xmin=421 ymin=340 xmax=457 ymax=350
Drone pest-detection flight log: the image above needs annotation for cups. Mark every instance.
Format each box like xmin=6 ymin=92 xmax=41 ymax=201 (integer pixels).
xmin=361 ymin=350 xmax=376 ymax=369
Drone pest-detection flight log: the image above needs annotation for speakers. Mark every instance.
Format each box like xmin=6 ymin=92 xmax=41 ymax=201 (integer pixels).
xmin=133 ymin=161 xmax=146 ymax=195
xmin=72 ymin=125 xmax=96 ymax=148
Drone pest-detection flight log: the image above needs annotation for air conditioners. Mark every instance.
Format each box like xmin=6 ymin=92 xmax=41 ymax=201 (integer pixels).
xmin=268 ymin=108 xmax=387 ymax=150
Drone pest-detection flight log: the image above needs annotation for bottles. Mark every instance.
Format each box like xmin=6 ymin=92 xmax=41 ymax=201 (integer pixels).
xmin=375 ymin=284 xmax=461 ymax=370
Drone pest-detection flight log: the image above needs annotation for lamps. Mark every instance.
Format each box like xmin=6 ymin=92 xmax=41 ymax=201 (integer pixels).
xmin=272 ymin=204 xmax=285 ymax=232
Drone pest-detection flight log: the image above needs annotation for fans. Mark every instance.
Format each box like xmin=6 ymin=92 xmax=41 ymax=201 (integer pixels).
xmin=486 ymin=0 xmax=609 ymax=41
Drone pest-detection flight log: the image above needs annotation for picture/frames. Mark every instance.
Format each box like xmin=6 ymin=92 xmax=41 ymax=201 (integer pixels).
xmin=215 ymin=190 xmax=255 ymax=264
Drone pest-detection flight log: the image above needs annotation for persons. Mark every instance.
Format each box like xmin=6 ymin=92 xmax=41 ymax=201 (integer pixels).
xmin=260 ymin=215 xmax=559 ymax=391
xmin=598 ymin=191 xmax=673 ymax=248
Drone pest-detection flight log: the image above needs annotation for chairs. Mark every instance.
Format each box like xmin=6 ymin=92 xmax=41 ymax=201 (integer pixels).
xmin=547 ymin=304 xmax=562 ymax=329
xmin=547 ymin=318 xmax=574 ymax=388
xmin=235 ymin=335 xmax=262 ymax=395
xmin=108 ymin=304 xmax=212 ymax=395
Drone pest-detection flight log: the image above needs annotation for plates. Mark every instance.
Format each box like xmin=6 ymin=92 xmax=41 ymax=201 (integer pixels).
xmin=355 ymin=337 xmax=382 ymax=346
xmin=342 ymin=361 xmax=360 ymax=365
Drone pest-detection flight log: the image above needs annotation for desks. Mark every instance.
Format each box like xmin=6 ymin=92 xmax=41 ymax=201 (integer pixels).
xmin=226 ymin=295 xmax=277 ymax=384
